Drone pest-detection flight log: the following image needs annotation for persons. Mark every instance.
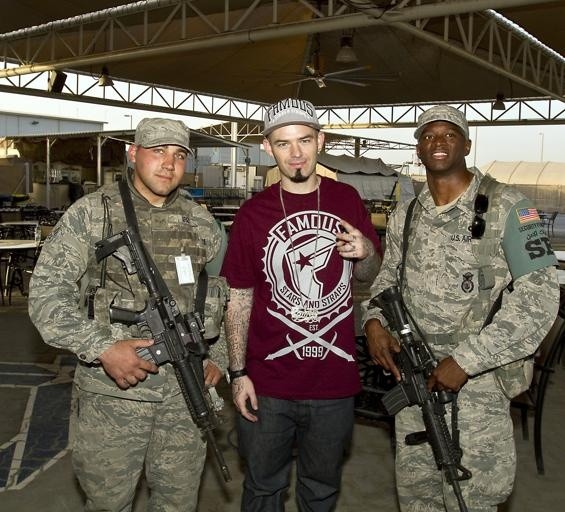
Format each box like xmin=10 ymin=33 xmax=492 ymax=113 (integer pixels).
xmin=28 ymin=117 xmax=230 ymax=512
xmin=361 ymin=106 xmax=561 ymax=512
xmin=218 ymin=97 xmax=384 ymax=512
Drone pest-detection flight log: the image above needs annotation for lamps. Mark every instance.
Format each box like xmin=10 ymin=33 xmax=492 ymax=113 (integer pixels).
xmin=334 ymin=36 xmax=357 ymax=62
xmin=490 ymin=75 xmax=505 ymax=110
xmin=98 ymin=65 xmax=111 ymax=86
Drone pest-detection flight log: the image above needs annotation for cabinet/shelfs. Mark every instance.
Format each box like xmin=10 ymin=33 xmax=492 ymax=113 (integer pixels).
xmin=0 ymin=201 xmax=240 ymax=308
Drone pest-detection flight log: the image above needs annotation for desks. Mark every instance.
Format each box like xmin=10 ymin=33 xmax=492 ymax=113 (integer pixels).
xmin=352 ymin=308 xmax=396 ymax=454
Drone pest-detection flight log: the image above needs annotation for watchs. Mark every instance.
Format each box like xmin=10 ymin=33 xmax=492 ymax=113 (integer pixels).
xmin=225 ymin=368 xmax=248 ymax=385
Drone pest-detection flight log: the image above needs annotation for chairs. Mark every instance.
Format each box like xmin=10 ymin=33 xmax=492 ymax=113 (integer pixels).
xmin=511 ymin=313 xmax=565 ymax=477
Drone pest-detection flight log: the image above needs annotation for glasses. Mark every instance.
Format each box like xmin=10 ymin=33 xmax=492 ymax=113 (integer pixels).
xmin=472 ymin=195 xmax=488 ymax=240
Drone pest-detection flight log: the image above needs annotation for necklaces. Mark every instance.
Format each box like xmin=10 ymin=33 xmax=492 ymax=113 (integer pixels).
xmin=279 ymin=179 xmax=320 ymax=324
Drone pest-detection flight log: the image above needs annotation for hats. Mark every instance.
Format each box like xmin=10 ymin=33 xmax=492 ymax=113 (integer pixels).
xmin=413 ymin=104 xmax=469 ymax=141
xmin=261 ymin=97 xmax=321 ymax=137
xmin=135 ymin=118 xmax=195 ymax=158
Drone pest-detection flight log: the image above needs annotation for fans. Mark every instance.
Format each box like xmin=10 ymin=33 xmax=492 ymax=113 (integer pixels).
xmin=277 ymin=51 xmax=374 ymax=92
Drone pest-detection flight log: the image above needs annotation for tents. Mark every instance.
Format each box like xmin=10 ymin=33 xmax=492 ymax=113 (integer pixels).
xmin=264 ymin=153 xmax=416 ymax=200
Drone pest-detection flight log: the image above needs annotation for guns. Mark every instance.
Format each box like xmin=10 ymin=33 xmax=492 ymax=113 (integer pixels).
xmin=368 ymin=286 xmax=472 ymax=511
xmin=94 ymin=226 xmax=232 ymax=482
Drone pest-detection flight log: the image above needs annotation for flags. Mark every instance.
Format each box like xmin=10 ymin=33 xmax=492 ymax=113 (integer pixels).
xmin=516 ymin=208 xmax=541 ymax=225
